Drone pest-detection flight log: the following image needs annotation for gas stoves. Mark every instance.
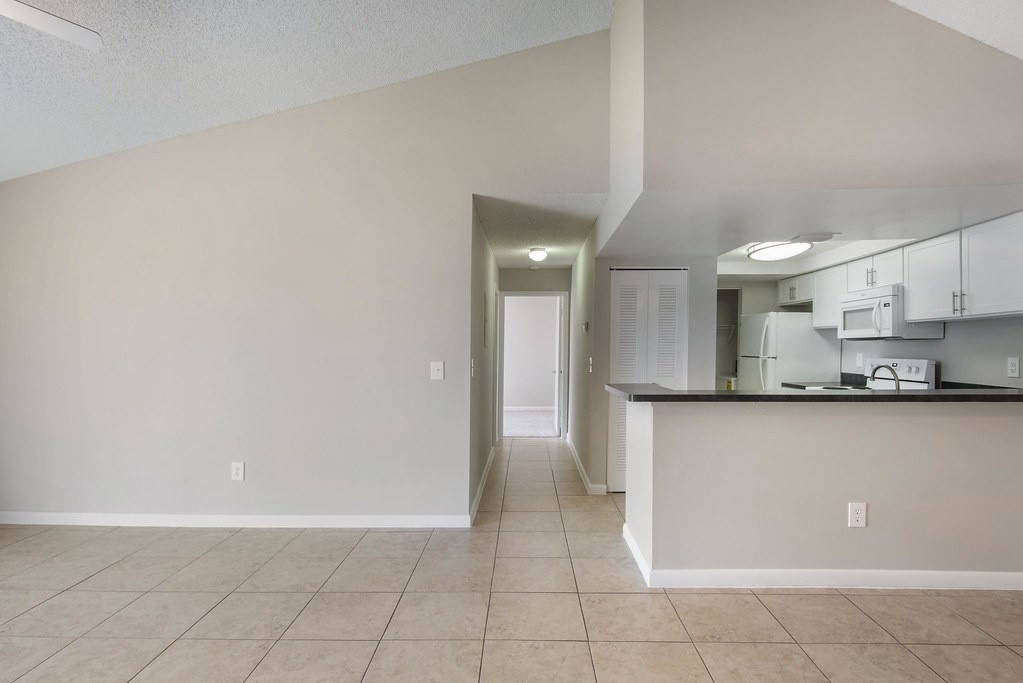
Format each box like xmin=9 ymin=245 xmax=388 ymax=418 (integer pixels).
xmin=824 ymin=357 xmax=936 ymax=389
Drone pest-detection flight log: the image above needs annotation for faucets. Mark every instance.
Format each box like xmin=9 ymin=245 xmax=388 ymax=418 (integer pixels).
xmin=869 ymin=364 xmax=901 ymax=391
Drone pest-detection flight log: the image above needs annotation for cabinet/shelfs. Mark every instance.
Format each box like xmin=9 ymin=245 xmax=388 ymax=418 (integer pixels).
xmin=776 ymin=271 xmax=815 ymax=307
xmin=846 ymin=245 xmax=904 ymax=293
xmin=903 ymin=209 xmax=1023 ymax=323
xmin=812 ymin=261 xmax=848 ymax=329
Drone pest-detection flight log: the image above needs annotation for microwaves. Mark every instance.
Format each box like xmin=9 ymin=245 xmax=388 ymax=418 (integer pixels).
xmin=837 ymin=284 xmax=944 ymax=340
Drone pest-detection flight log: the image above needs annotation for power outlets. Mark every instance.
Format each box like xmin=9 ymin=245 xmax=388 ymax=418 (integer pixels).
xmin=231 ymin=461 xmax=245 ymax=481
xmin=1006 ymin=357 xmax=1020 ymax=378
xmin=848 ymin=502 xmax=866 ymax=528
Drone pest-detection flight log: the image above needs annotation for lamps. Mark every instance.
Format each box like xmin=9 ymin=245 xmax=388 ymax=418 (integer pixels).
xmin=746 ymin=241 xmax=814 ymax=262
xmin=528 ymin=247 xmax=548 ymax=262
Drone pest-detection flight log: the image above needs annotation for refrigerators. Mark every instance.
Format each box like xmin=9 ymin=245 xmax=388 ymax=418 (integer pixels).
xmin=735 ymin=311 xmax=842 ymax=389
